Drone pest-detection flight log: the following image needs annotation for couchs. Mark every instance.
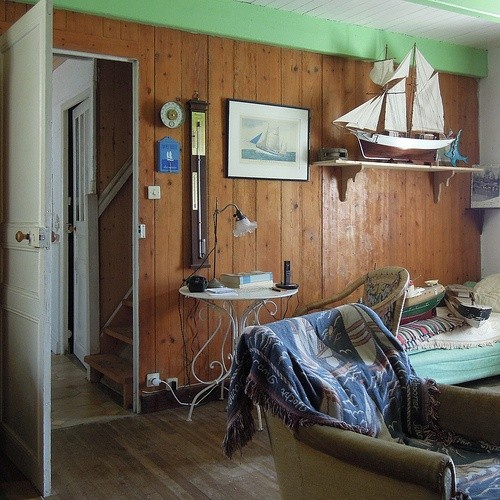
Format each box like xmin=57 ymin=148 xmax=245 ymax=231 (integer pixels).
xmin=243 ymin=302 xmax=499 ymax=499
xmin=400 ymin=272 xmax=499 ymax=385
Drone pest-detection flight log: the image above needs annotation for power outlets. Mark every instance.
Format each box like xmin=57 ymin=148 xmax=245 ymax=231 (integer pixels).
xmin=166 ymin=377 xmax=179 ymax=392
xmin=146 ymin=371 xmax=161 ymax=388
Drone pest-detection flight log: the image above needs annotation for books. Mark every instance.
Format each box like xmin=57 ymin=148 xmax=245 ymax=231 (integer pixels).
xmin=220 ymin=270 xmax=273 ymax=289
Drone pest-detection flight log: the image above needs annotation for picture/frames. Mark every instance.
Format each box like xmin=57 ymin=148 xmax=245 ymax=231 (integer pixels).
xmin=225 ymin=98 xmax=310 ymax=181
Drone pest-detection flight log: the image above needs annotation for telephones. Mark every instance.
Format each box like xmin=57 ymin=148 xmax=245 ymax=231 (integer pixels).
xmin=276 ymin=260 xmax=298 ymax=289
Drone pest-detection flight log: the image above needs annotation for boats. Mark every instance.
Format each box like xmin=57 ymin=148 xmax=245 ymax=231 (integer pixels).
xmin=443 ymin=282 xmax=493 ymax=328
xmin=398 ymin=277 xmax=446 ymax=325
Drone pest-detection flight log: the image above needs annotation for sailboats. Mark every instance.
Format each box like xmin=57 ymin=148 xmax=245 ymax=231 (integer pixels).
xmin=248 ymin=125 xmax=288 ymax=158
xmin=331 ymin=43 xmax=459 ymax=164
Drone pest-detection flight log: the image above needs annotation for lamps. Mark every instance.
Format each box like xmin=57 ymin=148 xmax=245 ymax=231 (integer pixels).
xmin=208 ymin=197 xmax=258 ymax=289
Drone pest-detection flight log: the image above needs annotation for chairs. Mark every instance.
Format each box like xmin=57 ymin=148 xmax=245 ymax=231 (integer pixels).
xmin=295 ymin=266 xmax=410 ymax=338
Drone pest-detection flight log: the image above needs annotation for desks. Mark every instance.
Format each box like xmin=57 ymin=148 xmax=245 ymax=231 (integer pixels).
xmin=180 ymin=282 xmax=298 ymax=431
